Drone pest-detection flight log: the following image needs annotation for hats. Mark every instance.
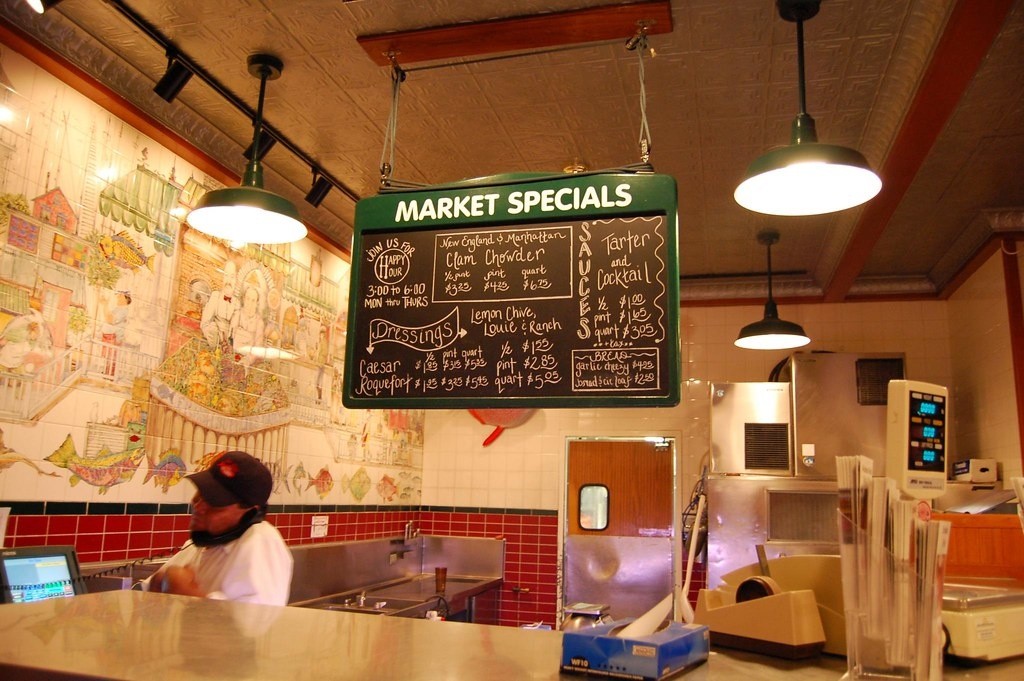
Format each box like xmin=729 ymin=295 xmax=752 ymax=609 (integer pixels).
xmin=183 ymin=450 xmax=273 ymax=507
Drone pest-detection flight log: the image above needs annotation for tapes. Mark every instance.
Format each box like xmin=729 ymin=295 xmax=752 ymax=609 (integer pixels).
xmin=735 ymin=575 xmax=780 ymax=603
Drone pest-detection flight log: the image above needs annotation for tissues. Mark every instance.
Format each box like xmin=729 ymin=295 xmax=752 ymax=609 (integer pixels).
xmin=558 ymin=594 xmax=712 ymax=681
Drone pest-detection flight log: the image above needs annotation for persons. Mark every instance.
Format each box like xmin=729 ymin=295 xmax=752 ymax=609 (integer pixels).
xmin=167 ymin=597 xmax=287 ymax=681
xmin=133 ymin=449 xmax=294 ymax=605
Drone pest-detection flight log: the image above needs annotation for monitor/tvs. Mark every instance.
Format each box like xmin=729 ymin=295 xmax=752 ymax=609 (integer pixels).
xmin=0 ymin=546 xmax=88 ymax=605
xmin=885 ymin=379 xmax=950 ymax=502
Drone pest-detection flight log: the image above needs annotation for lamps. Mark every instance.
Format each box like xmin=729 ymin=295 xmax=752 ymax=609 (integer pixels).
xmin=304 ymin=167 xmax=334 ymax=209
xmin=153 ymin=52 xmax=194 ymax=105
xmin=243 ymin=125 xmax=277 ymax=161
xmin=26 ymin=0 xmax=63 ymax=14
xmin=733 ymin=229 xmax=812 ymax=351
xmin=734 ymin=0 xmax=882 ymax=217
xmin=187 ymin=54 xmax=308 ymax=244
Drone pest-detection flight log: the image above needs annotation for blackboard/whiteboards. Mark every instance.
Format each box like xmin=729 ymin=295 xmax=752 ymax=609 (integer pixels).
xmin=350 ymin=213 xmax=669 ymax=398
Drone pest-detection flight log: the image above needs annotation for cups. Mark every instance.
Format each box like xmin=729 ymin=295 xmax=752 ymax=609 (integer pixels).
xmin=435 ymin=567 xmax=447 ymax=596
